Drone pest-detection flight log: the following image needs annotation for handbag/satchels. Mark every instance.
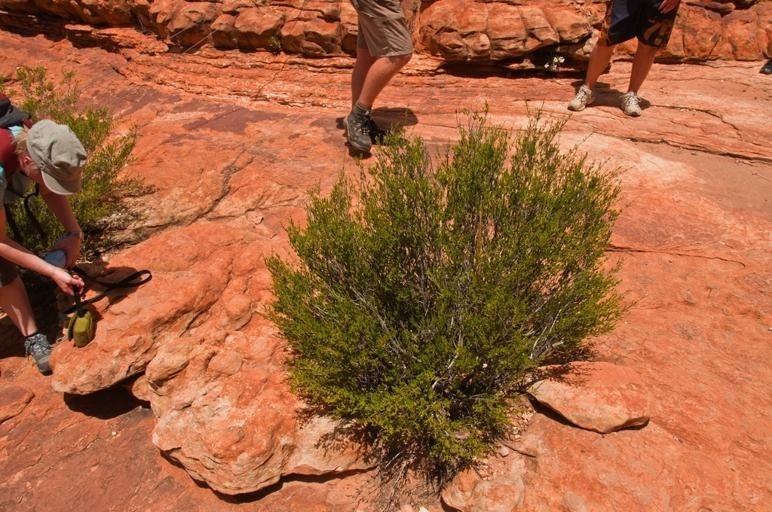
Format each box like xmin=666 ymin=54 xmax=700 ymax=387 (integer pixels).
xmin=68 ymin=309 xmax=92 ymax=347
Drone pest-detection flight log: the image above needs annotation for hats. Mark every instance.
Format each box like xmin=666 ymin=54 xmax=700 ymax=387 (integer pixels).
xmin=26 ymin=118 xmax=88 ymax=195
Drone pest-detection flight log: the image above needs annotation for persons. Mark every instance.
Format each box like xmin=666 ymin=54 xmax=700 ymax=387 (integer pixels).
xmin=342 ymin=0 xmax=414 ymax=158
xmin=0 ymin=89 xmax=90 ymax=376
xmin=563 ymin=0 xmax=678 ymax=117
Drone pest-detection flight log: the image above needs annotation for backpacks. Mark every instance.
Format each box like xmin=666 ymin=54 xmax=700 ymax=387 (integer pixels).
xmin=0 ymin=93 xmax=37 ymax=177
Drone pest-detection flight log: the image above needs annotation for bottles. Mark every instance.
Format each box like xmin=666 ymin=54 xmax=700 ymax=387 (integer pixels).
xmin=41 ymin=235 xmax=67 ymax=282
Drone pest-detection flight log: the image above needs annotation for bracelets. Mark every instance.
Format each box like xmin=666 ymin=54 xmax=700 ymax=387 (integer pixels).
xmin=70 ymin=230 xmax=85 ymax=241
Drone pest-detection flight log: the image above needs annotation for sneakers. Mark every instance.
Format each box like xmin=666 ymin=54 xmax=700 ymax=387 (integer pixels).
xmin=25 ymin=333 xmax=53 ymax=376
xmin=568 ymin=84 xmax=596 ymax=112
xmin=759 ymin=59 xmax=772 ymax=74
xmin=346 ymin=111 xmax=372 ymax=153
xmin=369 ymin=119 xmax=388 ymax=145
xmin=620 ymin=91 xmax=642 ymax=116
